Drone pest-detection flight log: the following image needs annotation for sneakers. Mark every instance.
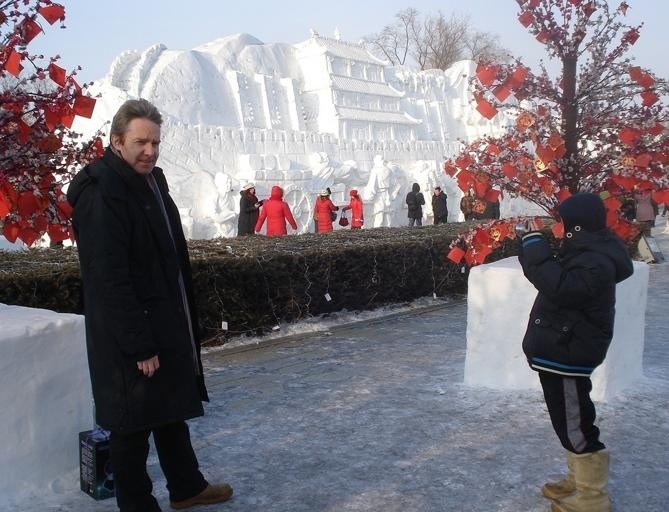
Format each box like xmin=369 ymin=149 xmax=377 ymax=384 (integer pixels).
xmin=170 ymin=484 xmax=233 ymax=509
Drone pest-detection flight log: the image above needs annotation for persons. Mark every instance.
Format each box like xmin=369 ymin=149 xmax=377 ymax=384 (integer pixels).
xmin=517 ymin=191 xmax=635 ymax=512
xmin=254 ymin=185 xmax=298 ymax=236
xmin=627 ymin=192 xmax=659 ymax=261
xmin=460 ymin=190 xmax=475 ymax=221
xmin=313 ymin=187 xmax=331 ymax=233
xmin=342 ymin=190 xmax=365 ymax=229
xmin=406 ymin=183 xmax=425 ymax=226
xmin=237 ymin=182 xmax=262 ymax=236
xmin=315 ymin=190 xmax=339 ymax=232
xmin=67 ymin=97 xmax=234 ymax=511
xmin=432 ymin=186 xmax=449 ymax=224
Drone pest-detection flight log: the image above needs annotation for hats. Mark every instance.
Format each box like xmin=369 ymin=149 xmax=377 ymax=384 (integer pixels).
xmin=243 ymin=182 xmax=254 ymax=191
xmin=350 ymin=190 xmax=357 ymax=196
xmin=321 ymin=189 xmax=329 ymax=196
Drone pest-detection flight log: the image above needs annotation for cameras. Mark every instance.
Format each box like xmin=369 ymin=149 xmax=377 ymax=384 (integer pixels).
xmin=515 ymin=221 xmax=531 ymax=234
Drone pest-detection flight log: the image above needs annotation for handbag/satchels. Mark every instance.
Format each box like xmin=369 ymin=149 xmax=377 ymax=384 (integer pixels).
xmin=339 ymin=217 xmax=349 ymax=226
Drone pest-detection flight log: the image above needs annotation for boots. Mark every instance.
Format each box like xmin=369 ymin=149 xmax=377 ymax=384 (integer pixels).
xmin=541 ymin=449 xmax=610 ymax=512
